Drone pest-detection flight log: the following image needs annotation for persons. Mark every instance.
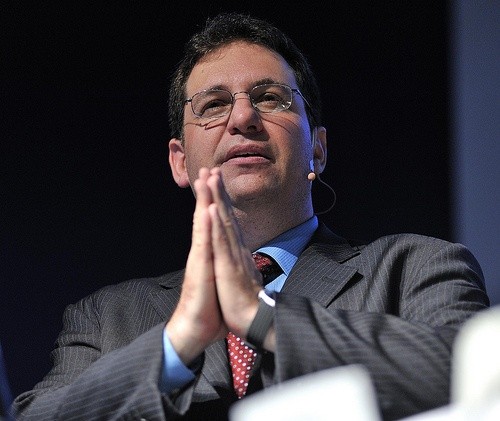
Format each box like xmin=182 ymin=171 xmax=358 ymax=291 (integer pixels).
xmin=3 ymin=16 xmax=491 ymax=421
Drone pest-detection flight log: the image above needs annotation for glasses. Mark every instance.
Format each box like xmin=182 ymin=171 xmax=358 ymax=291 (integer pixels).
xmin=173 ymin=81 xmax=312 ymax=120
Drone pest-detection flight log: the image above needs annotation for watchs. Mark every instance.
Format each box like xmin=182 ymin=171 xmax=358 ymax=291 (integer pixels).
xmin=243 ymin=288 xmax=280 ymax=352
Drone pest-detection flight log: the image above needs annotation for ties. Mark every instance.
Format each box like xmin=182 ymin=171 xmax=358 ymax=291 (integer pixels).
xmin=226 ymin=253 xmax=273 ymax=399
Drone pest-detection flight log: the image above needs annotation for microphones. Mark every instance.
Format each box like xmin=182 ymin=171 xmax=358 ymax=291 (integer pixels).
xmin=307 ymin=171 xmax=322 ymax=185
